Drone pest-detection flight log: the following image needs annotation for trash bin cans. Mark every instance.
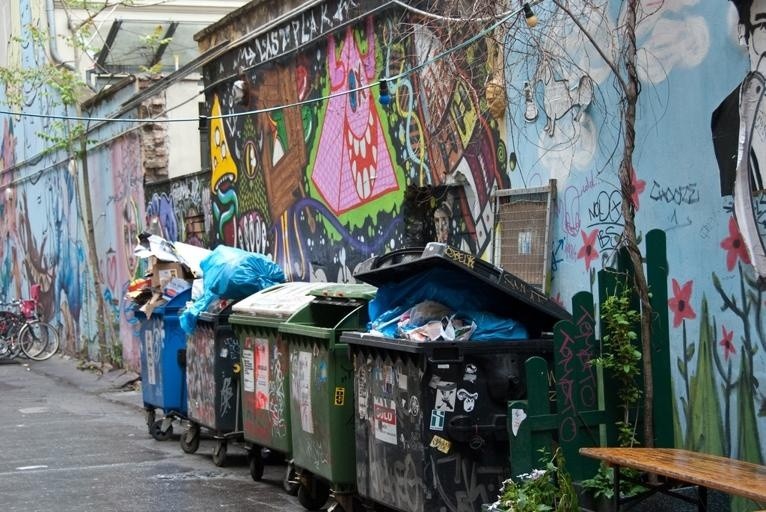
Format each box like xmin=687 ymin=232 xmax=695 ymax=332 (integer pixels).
xmin=279 ymin=284 xmax=377 ymax=512
xmin=228 ymin=281 xmax=327 ymax=496
xmin=131 ymin=286 xmax=191 ymax=441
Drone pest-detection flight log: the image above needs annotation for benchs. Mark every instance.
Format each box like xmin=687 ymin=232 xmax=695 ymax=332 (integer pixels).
xmin=580 ymin=447 xmax=766 ymax=510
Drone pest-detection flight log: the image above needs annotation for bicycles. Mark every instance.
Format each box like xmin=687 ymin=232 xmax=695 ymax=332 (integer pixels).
xmin=1 ymin=300 xmax=59 ymax=360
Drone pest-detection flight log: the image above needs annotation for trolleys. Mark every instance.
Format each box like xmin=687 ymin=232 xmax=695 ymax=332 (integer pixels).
xmin=229 ymin=282 xmax=292 ymax=481
xmin=277 ymin=284 xmax=355 ymax=509
xmin=135 ymin=288 xmax=187 ymax=440
